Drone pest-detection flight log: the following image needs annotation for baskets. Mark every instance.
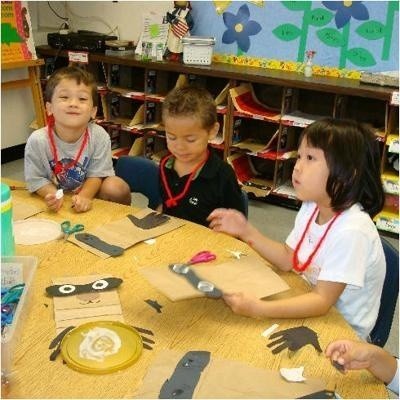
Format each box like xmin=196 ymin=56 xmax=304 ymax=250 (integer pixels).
xmin=181 ymin=36 xmax=216 ymax=66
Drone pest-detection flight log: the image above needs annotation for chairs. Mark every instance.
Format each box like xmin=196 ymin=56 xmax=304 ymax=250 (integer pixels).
xmin=367 ymin=237 xmax=399 ymax=347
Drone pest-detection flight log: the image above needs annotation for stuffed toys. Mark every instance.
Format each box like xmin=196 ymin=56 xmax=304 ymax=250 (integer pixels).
xmin=161 ymin=1 xmax=194 ymax=62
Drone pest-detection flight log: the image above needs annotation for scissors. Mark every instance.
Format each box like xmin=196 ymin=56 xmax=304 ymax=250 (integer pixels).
xmin=181 ymin=250 xmax=216 ymax=270
xmin=0 ymin=283 xmax=25 ymax=333
xmin=55 ymin=221 xmax=84 ymax=244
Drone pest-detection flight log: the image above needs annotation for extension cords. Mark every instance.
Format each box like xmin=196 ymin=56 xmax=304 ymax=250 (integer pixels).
xmin=60 ymin=29 xmax=71 ymax=34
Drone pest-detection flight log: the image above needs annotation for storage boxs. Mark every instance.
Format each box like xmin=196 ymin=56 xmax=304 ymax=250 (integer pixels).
xmin=0 ymin=255 xmax=39 ymax=375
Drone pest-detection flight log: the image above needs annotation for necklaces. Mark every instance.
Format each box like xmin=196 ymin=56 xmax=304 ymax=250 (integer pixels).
xmin=291 ymin=205 xmax=344 ymax=275
xmin=45 ymin=118 xmax=91 ymax=176
xmin=158 ymin=151 xmax=211 ymax=207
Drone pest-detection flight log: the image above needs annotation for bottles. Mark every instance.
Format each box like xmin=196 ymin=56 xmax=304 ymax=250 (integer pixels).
xmin=141 ymin=41 xmax=164 ymax=63
xmin=1 ymin=183 xmax=18 ymax=255
xmin=303 ymin=56 xmax=313 ymax=78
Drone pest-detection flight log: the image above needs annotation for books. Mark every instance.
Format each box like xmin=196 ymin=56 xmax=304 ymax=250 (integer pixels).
xmin=44 ymin=57 xmax=399 ymax=238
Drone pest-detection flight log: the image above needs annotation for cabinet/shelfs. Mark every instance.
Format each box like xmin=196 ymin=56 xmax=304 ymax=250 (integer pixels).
xmin=35 ymin=44 xmax=399 ymax=235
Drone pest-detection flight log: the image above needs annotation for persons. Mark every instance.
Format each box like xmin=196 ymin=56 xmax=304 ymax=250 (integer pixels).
xmin=23 ymin=66 xmax=133 ymax=215
xmin=148 ymin=83 xmax=248 ymax=231
xmin=204 ymin=116 xmax=388 ymax=342
xmin=325 ymin=339 xmax=399 ymax=399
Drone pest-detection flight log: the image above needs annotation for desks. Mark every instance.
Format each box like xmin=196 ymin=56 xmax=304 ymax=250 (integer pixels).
xmin=0 ymin=176 xmax=391 ymax=399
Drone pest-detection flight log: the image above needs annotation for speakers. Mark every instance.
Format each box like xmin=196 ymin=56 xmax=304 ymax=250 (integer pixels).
xmin=47 ymin=32 xmax=118 ymax=53
